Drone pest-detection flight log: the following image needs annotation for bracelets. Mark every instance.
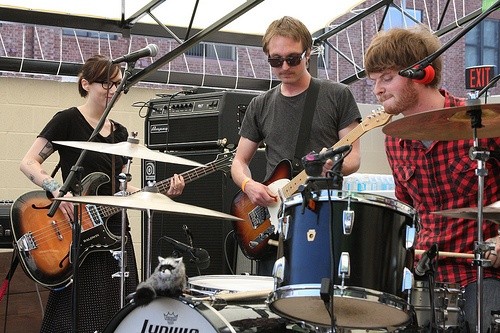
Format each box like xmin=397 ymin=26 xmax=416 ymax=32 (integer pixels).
xmin=241 ymin=178 xmax=252 ymax=193
xmin=42 ymin=178 xmax=61 ymax=192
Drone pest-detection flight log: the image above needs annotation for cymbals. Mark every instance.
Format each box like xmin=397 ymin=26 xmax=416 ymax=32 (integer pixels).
xmin=49 ymin=187 xmax=246 ymax=222
xmin=428 ymin=201 xmax=500 ymax=226
xmin=52 ymin=138 xmax=212 ymax=170
xmin=381 ymin=104 xmax=500 ymax=142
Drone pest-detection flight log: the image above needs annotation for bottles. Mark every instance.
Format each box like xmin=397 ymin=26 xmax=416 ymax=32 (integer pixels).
xmin=343 ymin=173 xmax=395 ymax=191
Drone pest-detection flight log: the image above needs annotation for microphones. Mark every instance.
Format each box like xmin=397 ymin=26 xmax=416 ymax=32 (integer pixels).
xmin=163 ymin=236 xmax=211 ymax=270
xmin=415 ymin=243 xmax=437 ymax=276
xmin=111 ymin=43 xmax=159 ymax=64
xmin=397 ymin=63 xmax=435 ymax=86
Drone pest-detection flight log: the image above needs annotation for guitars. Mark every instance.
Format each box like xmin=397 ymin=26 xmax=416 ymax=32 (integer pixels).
xmin=229 ymin=103 xmax=392 ymax=262
xmin=8 ymin=145 xmax=243 ymax=289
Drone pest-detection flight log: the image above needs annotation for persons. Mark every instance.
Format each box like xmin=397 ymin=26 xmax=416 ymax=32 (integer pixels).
xmin=364 ymin=25 xmax=500 ymax=333
xmin=231 ymin=16 xmax=363 ymax=277
xmin=19 ymin=54 xmax=185 ymax=333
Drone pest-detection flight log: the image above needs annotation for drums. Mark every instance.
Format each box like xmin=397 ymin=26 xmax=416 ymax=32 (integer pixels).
xmin=187 ymin=272 xmax=280 ymax=306
xmin=406 ymin=282 xmax=463 ymax=329
xmin=99 ymin=290 xmax=292 ymax=333
xmin=266 ymin=186 xmax=420 ymax=332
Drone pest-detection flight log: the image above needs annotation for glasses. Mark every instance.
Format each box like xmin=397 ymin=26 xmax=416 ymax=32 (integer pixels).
xmin=267 ymin=48 xmax=308 ymax=68
xmin=91 ymin=80 xmax=122 ymax=89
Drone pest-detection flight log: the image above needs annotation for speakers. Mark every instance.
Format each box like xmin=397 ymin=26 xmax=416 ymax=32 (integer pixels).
xmin=139 ymin=148 xmax=270 ymax=290
